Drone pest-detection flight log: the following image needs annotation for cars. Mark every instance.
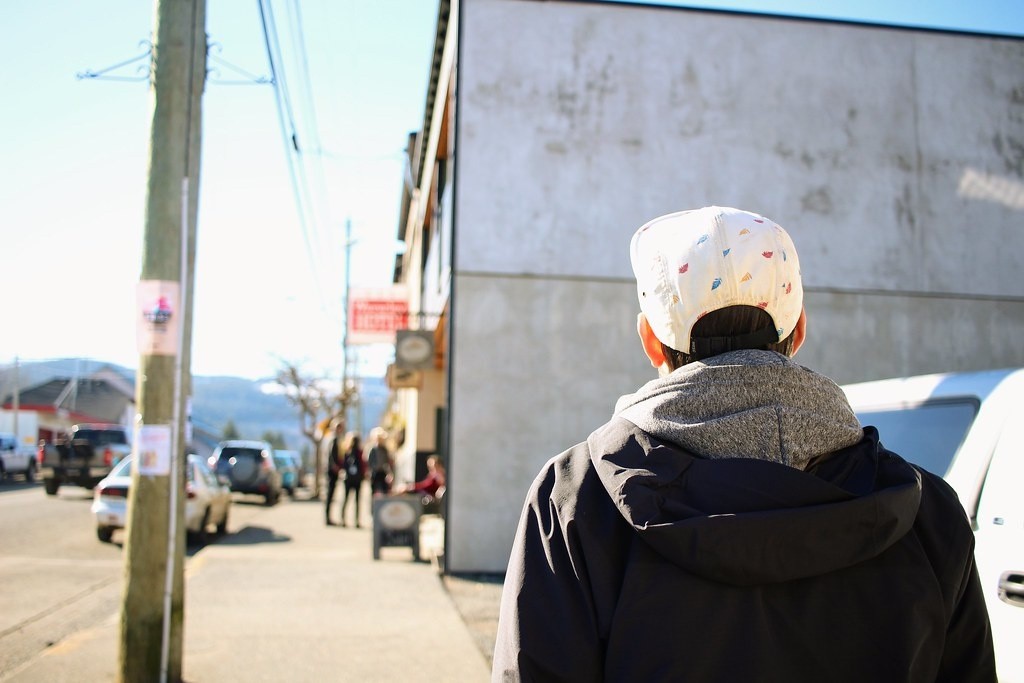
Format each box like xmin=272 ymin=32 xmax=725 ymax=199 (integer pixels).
xmin=277 ymin=455 xmax=300 ymax=491
xmin=92 ymin=448 xmax=231 ymax=549
xmin=836 ymin=364 xmax=1024 ymax=683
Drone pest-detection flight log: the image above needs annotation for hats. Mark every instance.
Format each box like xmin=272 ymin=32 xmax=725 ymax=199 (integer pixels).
xmin=629 ymin=205 xmax=804 ymax=356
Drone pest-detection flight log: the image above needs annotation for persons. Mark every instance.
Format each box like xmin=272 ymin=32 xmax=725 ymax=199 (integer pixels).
xmin=321 ymin=416 xmax=396 ymax=528
xmin=485 ymin=204 xmax=999 ymax=683
xmin=397 ymin=453 xmax=448 ymax=512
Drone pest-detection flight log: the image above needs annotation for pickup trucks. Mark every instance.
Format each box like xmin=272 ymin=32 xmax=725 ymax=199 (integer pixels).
xmin=0 ymin=432 xmax=38 ymax=485
xmin=40 ymin=423 xmax=131 ymax=495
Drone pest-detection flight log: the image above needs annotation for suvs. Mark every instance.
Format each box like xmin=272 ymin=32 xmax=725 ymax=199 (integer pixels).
xmin=208 ymin=440 xmax=281 ymax=507
